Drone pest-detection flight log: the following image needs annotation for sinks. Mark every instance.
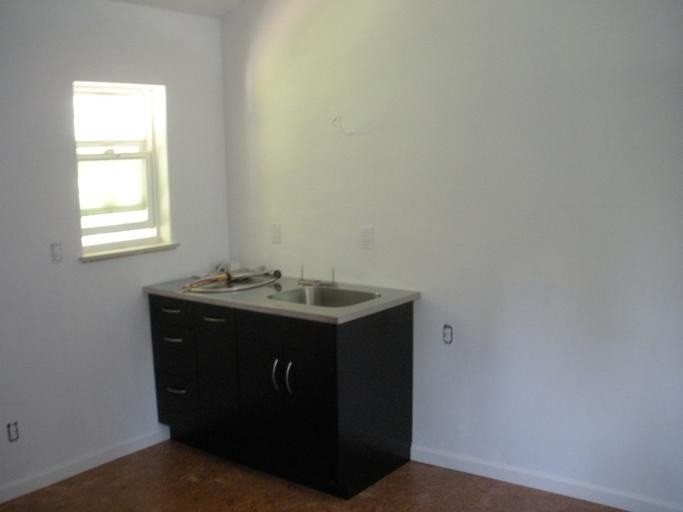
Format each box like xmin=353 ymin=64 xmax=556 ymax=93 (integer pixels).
xmin=268 ymin=286 xmax=376 ymax=308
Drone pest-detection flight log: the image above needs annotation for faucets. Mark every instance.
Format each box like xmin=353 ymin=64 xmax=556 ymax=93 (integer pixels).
xmin=318 ymin=282 xmax=332 ymax=286
xmin=297 ymin=278 xmax=315 ymax=285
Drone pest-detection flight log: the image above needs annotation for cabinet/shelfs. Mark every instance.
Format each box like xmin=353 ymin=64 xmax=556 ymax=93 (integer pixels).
xmin=235 ymin=301 xmax=413 ymax=499
xmin=148 ymin=294 xmax=240 ymax=471
